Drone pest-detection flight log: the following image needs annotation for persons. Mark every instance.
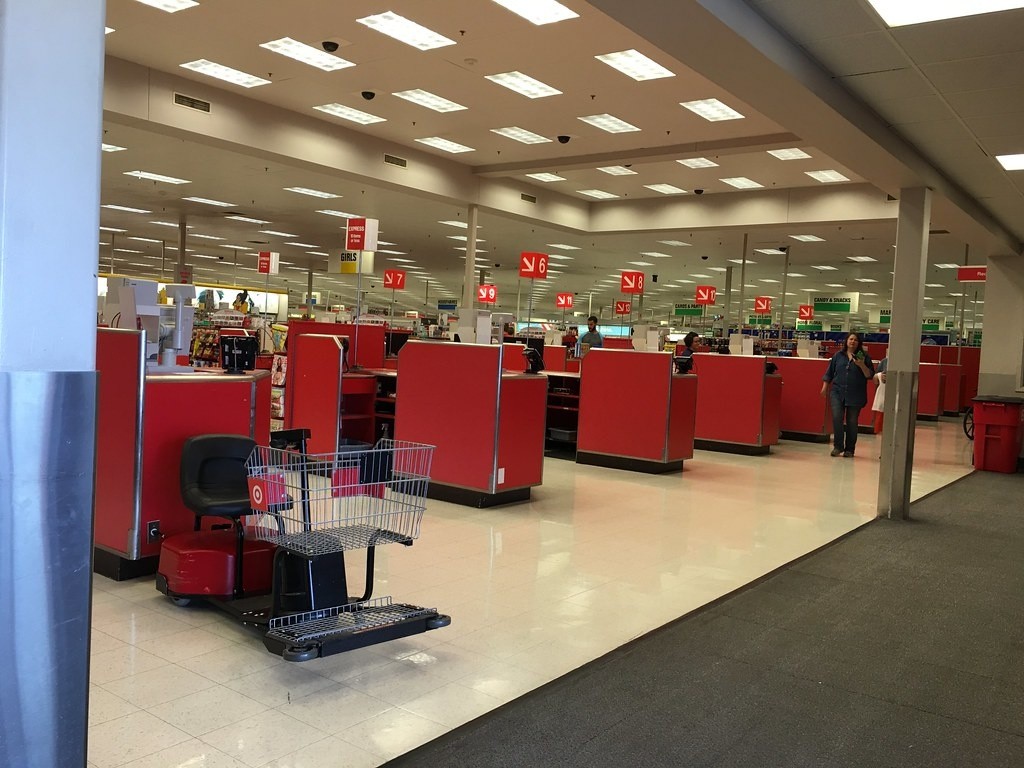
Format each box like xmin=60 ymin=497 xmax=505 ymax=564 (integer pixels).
xmin=873 ymin=358 xmax=889 ymax=459
xmin=821 ymin=332 xmax=874 ymax=457
xmin=576 ymin=316 xmax=602 ymax=348
xmin=678 ymin=332 xmax=700 ymax=372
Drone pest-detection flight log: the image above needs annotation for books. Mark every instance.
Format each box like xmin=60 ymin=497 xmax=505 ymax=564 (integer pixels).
xmin=271 ymin=387 xmax=285 ymax=419
xmin=271 ymin=354 xmax=287 ymax=385
xmin=270 ymin=419 xmax=286 ymax=433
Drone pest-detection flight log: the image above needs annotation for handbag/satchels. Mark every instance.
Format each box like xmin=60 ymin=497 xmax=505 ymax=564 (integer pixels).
xmin=871 ymin=372 xmax=885 ymax=413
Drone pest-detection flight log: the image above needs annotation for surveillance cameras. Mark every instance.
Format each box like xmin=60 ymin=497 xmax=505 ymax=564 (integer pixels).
xmin=495 ymin=264 xmax=500 ymax=267
xmin=779 ymin=248 xmax=786 ymax=251
xmin=694 ymin=189 xmax=703 ymax=194
xmin=362 ymin=92 xmax=375 ymax=100
xmin=321 ymin=42 xmax=338 ymax=52
xmin=702 ymin=256 xmax=708 ymax=260
xmin=558 ymin=136 xmax=570 ymax=143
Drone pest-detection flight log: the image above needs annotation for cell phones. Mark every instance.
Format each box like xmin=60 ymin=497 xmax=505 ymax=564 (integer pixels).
xmin=851 ymin=353 xmax=857 ymax=360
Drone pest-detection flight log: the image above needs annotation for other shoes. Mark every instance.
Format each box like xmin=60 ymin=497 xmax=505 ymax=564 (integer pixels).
xmin=831 ymin=447 xmax=844 ymax=456
xmin=843 ymin=450 xmax=854 ymax=457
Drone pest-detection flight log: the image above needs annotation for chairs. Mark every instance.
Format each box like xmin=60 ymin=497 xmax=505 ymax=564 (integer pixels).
xmin=180 ymin=435 xmax=294 ymax=601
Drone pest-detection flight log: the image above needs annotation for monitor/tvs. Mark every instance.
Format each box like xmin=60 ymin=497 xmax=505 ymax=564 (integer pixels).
xmin=220 ymin=335 xmax=257 ymax=371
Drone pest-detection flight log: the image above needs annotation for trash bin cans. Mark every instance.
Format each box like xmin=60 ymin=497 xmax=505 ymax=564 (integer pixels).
xmin=973 ymin=395 xmax=1024 ymax=474
